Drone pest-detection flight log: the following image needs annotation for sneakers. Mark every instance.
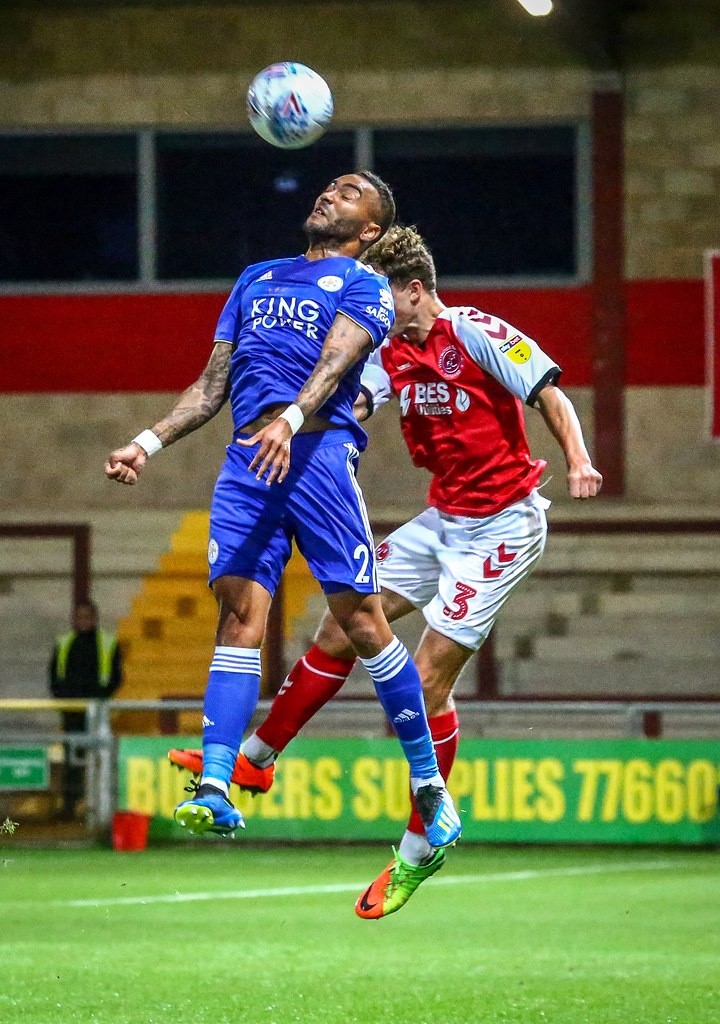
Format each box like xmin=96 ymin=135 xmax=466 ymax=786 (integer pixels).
xmin=411 ymin=773 xmax=463 ymax=850
xmin=356 ymin=843 xmax=446 ymax=920
xmin=172 ymin=780 xmax=245 ymax=840
xmin=168 ymin=749 xmax=276 ymax=798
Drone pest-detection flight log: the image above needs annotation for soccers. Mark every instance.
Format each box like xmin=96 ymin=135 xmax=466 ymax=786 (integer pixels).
xmin=245 ymin=62 xmax=333 ymax=150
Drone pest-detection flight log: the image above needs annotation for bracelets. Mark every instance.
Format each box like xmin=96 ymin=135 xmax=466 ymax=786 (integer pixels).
xmin=278 ymin=404 xmax=305 ymax=435
xmin=129 ymin=428 xmax=163 ymax=459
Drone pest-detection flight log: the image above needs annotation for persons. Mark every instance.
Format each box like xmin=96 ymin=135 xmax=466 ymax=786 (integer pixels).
xmin=164 ymin=225 xmax=602 ymax=921
xmin=46 ymin=597 xmax=124 ymax=825
xmin=104 ymin=169 xmax=465 ymax=852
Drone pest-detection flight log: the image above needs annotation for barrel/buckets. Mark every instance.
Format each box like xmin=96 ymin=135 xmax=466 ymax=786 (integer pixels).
xmin=111 ymin=811 xmax=148 ymax=852
